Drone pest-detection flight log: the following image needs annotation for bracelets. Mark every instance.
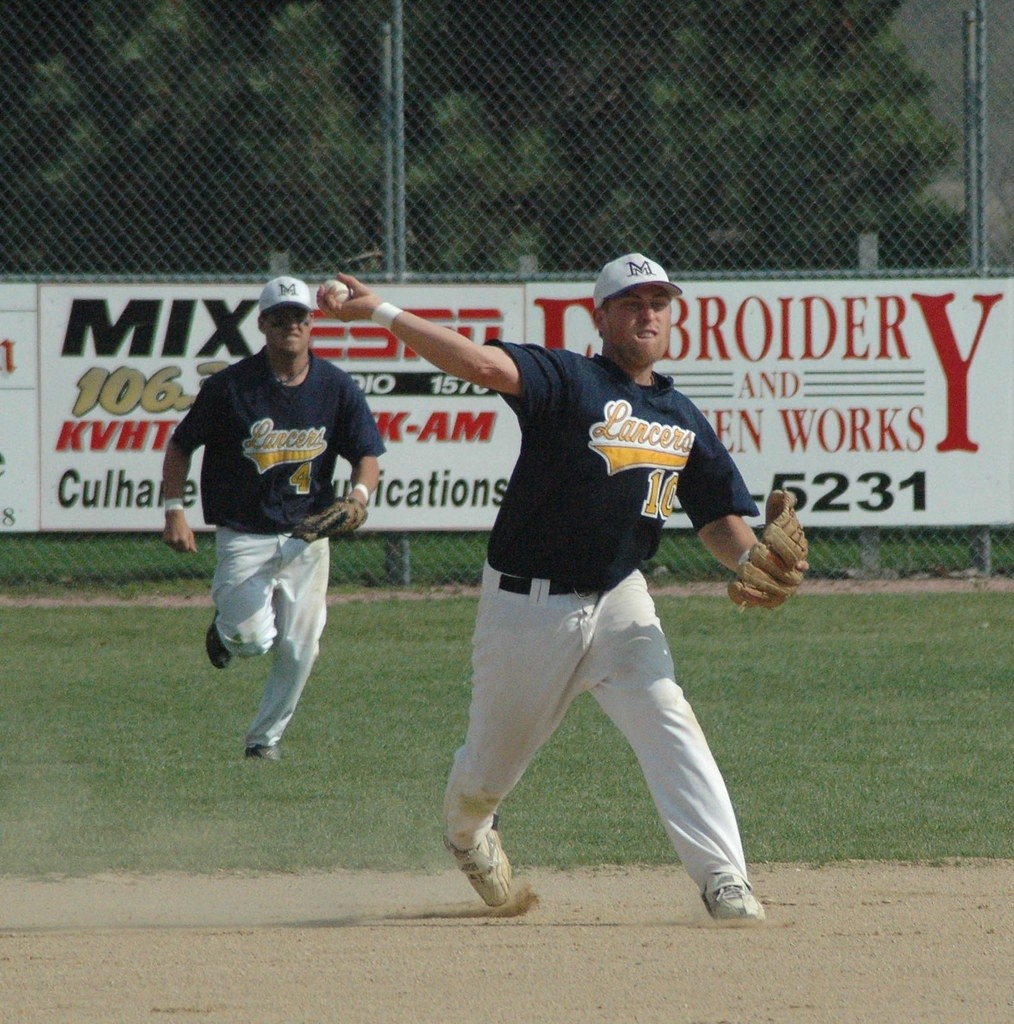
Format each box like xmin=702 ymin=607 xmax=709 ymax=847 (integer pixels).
xmin=355 ymin=484 xmax=368 ymax=500
xmin=736 ymin=549 xmax=750 ymax=567
xmin=165 ymin=498 xmax=183 ymax=512
xmin=372 ymin=303 xmax=402 ymax=328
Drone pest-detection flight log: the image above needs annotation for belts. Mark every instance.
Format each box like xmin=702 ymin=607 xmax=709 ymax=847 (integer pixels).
xmin=497 ymin=571 xmax=602 ymax=599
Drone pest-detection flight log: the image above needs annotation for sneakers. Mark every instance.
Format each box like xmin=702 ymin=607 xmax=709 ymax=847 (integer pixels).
xmin=447 ymin=813 xmax=513 ymax=906
xmin=701 ymin=872 xmax=764 ymax=922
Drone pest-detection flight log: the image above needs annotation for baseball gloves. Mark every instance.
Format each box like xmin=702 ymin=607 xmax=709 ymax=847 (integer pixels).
xmin=291 ymin=495 xmax=369 ymax=543
xmin=726 ymin=487 xmax=811 ymax=609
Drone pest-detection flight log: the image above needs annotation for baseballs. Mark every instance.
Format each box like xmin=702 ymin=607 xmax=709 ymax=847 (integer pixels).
xmin=323 ymin=278 xmax=350 ymax=304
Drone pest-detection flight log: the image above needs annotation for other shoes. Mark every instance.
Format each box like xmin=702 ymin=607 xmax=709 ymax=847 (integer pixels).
xmin=246 ymin=745 xmax=281 ymax=762
xmin=204 ymin=608 xmax=233 ymax=668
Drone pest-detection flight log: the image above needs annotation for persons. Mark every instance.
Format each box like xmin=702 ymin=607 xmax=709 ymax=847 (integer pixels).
xmin=160 ymin=274 xmax=387 ymax=761
xmin=317 ymin=253 xmax=809 ymax=924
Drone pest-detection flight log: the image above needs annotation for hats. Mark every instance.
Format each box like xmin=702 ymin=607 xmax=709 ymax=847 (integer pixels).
xmin=594 ymin=253 xmax=682 ymax=310
xmin=258 ymin=275 xmax=313 ymax=316
